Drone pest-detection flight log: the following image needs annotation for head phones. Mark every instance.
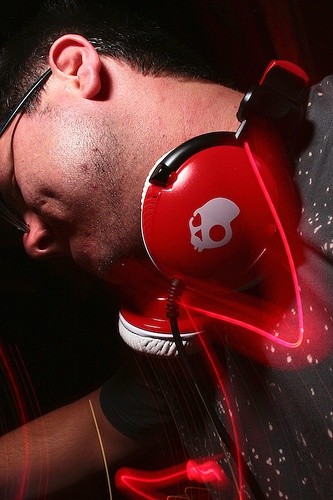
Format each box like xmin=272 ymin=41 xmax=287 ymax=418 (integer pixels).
xmin=116 ymin=61 xmax=314 ymax=354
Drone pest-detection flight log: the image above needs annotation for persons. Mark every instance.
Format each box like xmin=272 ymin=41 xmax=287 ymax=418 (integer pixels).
xmin=1 ymin=0 xmax=333 ymax=500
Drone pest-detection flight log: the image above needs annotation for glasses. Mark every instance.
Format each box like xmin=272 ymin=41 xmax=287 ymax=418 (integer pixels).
xmin=0 ymin=66 xmax=53 ymax=232
